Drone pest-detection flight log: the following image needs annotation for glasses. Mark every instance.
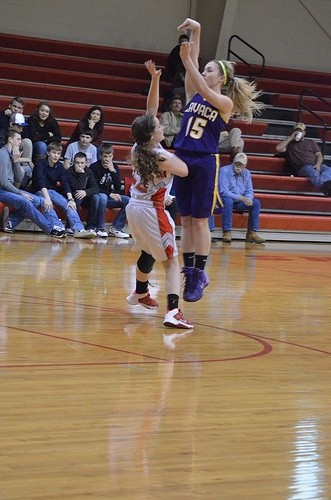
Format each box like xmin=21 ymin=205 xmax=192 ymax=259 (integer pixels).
xmin=235 ymin=162 xmax=244 ymax=167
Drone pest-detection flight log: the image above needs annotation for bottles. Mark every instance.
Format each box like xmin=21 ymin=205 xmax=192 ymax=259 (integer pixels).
xmin=120 ymin=180 xmax=125 ymax=194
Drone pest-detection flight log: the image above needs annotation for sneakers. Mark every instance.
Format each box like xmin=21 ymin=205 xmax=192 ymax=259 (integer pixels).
xmin=125 ymin=290 xmax=159 ymax=311
xmin=180 ymin=266 xmax=198 ymax=301
xmin=162 ymin=308 xmax=195 ymax=329
xmin=184 ymin=268 xmax=208 ymax=302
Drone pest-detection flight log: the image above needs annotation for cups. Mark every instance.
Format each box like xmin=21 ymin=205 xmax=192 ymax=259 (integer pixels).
xmin=295 ymin=132 xmax=302 ymax=141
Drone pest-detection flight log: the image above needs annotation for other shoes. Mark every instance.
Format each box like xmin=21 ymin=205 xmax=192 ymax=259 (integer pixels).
xmin=108 ymin=225 xmax=130 ymax=238
xmin=50 ymin=227 xmax=67 ymax=238
xmin=246 ymin=231 xmax=266 ymax=244
xmin=64 ymin=227 xmax=74 ymax=237
xmin=222 ymin=231 xmax=232 ymax=242
xmin=95 ymin=229 xmax=109 ymax=237
xmin=73 ymin=228 xmax=96 ymax=239
xmin=3 ymin=216 xmax=15 ymax=234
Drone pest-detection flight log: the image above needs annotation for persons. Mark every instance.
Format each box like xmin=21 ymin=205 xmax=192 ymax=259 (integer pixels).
xmin=66 ymin=106 xmax=105 ymax=146
xmin=159 ymin=94 xmax=186 ymax=149
xmin=218 ymin=127 xmax=244 ymax=162
xmin=218 ymin=153 xmax=266 ymax=244
xmin=0 ymin=97 xmax=61 ymax=187
xmin=150 ymin=18 xmax=273 ymax=302
xmin=32 ymin=142 xmax=97 ymax=238
xmin=64 ymin=126 xmax=131 ymax=238
xmin=166 ymin=34 xmax=189 ymax=88
xmin=275 ymin=122 xmax=331 ymax=198
xmin=0 ymin=129 xmax=67 ymax=237
xmin=126 ymin=59 xmax=194 ymax=329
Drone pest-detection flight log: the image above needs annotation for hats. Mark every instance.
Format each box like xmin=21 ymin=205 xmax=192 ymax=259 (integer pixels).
xmin=8 ymin=113 xmax=29 ymax=126
xmin=233 ymin=152 xmax=248 ymax=165
xmin=293 ymin=123 xmax=305 ymax=131
xmin=167 ymin=94 xmax=185 ymax=104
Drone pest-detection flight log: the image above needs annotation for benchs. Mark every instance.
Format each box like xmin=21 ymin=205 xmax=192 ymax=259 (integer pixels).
xmin=0 ymin=33 xmax=331 ymax=242
